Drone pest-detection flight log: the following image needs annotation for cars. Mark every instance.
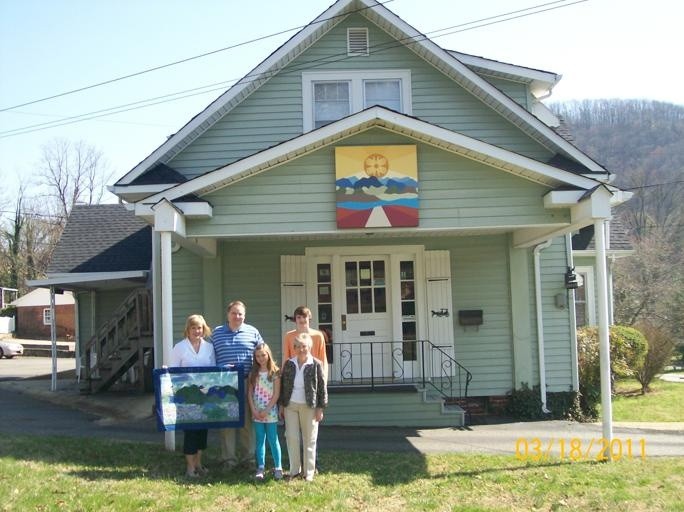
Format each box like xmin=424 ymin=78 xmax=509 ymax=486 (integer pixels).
xmin=0 ymin=339 xmax=24 ymax=360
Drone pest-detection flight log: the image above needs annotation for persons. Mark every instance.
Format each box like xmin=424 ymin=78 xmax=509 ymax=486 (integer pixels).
xmin=282 ymin=306 xmax=328 ymax=473
xmin=248 ymin=343 xmax=284 ymax=480
xmin=161 ymin=315 xmax=234 ymax=477
xmin=277 ymin=333 xmax=328 ymax=482
xmin=208 ymin=299 xmax=264 ymax=473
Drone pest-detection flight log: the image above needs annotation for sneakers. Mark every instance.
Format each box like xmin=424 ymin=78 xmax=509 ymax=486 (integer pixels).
xmin=195 ymin=465 xmax=212 ymax=477
xmin=273 ymin=468 xmax=284 ymax=479
xmin=304 ymin=474 xmax=315 ymax=483
xmin=255 ymin=468 xmax=265 ymax=480
xmin=184 ymin=468 xmax=202 ymax=479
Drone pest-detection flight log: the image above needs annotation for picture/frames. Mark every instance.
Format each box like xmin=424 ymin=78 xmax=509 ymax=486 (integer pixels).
xmin=151 ymin=363 xmax=247 ymax=431
